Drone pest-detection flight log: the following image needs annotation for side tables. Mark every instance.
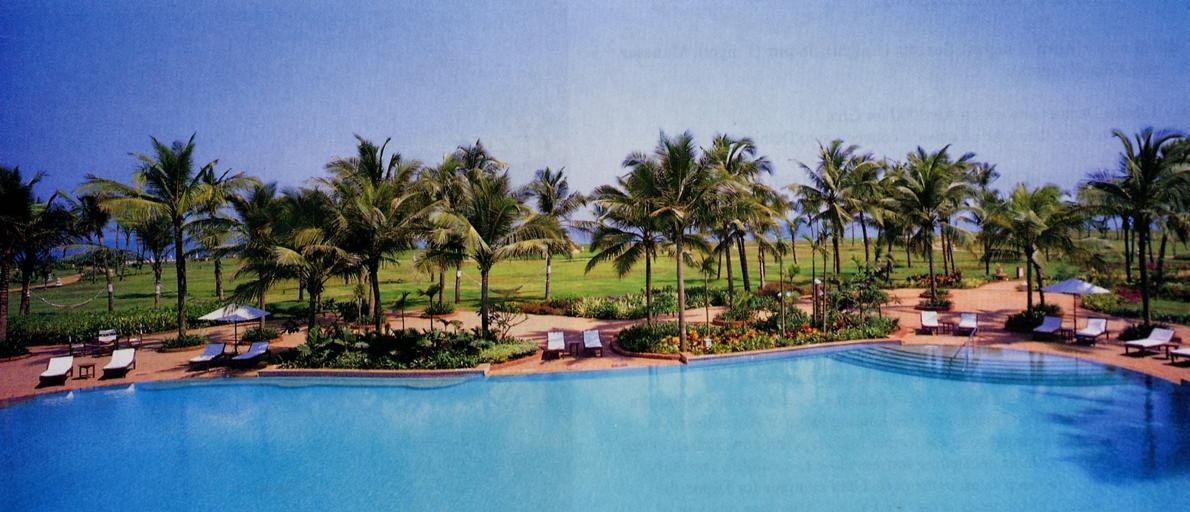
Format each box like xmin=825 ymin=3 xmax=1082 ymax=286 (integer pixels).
xmin=78 ymin=363 xmax=96 ymax=379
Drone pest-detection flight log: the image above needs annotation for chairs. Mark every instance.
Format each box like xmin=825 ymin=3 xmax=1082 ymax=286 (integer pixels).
xmin=1033 ymin=315 xmax=1190 ymax=365
xmin=39 ymin=354 xmax=73 ymax=385
xmin=189 ymin=340 xmax=269 ymax=371
xmin=102 ymin=348 xmax=136 ymax=378
xmin=920 ymin=310 xmax=978 ymax=336
xmin=68 ymin=327 xmax=143 ymax=356
xmin=547 ymin=329 xmax=603 ymax=360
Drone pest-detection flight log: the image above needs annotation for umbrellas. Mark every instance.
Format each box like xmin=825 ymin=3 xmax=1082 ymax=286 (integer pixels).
xmin=1041 ymin=276 xmax=1111 ymax=335
xmin=196 ymin=302 xmax=271 ymax=354
